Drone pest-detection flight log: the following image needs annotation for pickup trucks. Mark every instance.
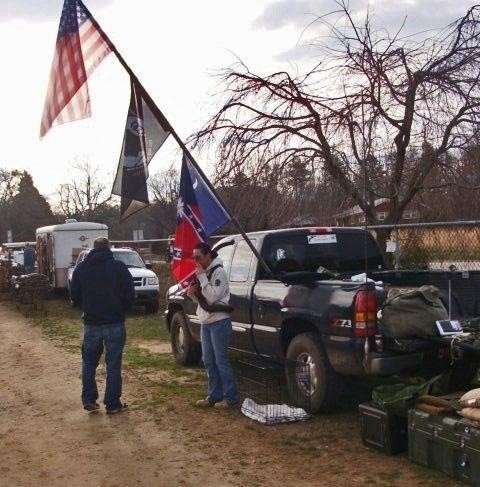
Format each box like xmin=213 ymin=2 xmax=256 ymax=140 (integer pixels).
xmin=163 ymin=226 xmax=480 ymax=415
xmin=67 ymin=247 xmax=161 ymax=315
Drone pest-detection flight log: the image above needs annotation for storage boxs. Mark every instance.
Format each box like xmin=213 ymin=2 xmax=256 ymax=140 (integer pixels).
xmin=408 ymin=392 xmax=480 ymax=487
xmin=359 ymin=400 xmax=408 ymax=456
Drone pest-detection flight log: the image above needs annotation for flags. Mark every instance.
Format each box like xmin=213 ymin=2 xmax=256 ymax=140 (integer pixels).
xmin=40 ymin=0 xmax=112 ymax=140
xmin=169 ymin=156 xmax=233 ymax=283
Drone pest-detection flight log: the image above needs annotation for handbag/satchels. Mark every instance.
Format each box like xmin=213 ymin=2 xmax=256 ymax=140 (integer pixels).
xmin=381 ymin=285 xmax=449 ymax=337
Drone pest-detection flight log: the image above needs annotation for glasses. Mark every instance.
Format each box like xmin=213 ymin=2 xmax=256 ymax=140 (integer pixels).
xmin=192 ymin=255 xmax=201 ymax=260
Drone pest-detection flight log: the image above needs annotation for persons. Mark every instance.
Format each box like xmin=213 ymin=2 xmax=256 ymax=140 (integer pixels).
xmin=110 ymin=76 xmax=171 ymax=224
xmin=181 ymin=244 xmax=240 ymax=407
xmin=70 ymin=236 xmax=135 ymax=413
xmin=0 ymin=241 xmax=35 ymax=274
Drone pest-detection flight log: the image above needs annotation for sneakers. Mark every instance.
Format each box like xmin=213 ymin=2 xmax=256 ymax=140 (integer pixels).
xmin=195 ymin=399 xmax=241 ymax=409
xmin=83 ymin=402 xmax=128 ymax=413
